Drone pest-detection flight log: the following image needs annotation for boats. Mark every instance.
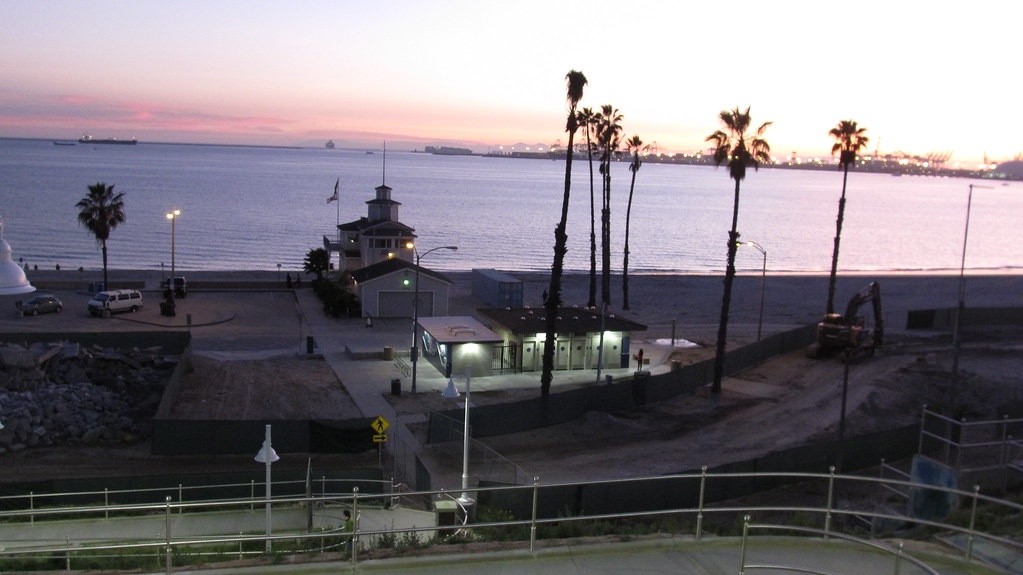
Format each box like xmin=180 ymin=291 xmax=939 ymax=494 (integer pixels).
xmin=53 ymin=141 xmax=76 ymax=145
xmin=79 ymin=133 xmax=137 ymax=145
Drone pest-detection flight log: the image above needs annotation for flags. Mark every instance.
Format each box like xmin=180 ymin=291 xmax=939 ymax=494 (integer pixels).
xmin=327 ymin=179 xmax=339 ymax=204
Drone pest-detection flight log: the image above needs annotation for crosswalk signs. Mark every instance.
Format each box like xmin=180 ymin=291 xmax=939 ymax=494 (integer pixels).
xmin=371 ymin=416 xmax=390 ymax=435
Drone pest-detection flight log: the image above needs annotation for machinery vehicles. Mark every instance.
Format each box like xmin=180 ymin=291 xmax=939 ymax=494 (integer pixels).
xmin=818 ymin=280 xmax=884 ymax=353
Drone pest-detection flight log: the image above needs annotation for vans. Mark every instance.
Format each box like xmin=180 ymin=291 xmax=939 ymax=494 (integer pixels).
xmin=88 ymin=289 xmax=143 ymax=317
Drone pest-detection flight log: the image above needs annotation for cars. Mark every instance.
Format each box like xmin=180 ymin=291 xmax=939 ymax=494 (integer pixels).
xmin=22 ymin=295 xmax=63 ymax=317
xmin=164 ymin=276 xmax=188 ymax=298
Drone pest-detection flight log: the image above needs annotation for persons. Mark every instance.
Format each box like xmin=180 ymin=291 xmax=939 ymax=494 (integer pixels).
xmin=342 ymin=510 xmax=353 ymax=560
xmin=638 ymin=349 xmax=643 ymax=371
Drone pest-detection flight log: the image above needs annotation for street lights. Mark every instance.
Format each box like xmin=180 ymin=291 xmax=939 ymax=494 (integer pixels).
xmin=441 ymin=364 xmax=471 ymax=500
xmin=736 ymin=240 xmax=767 ymax=342
xmin=254 ymin=425 xmax=280 ymax=555
xmin=167 ymin=210 xmax=180 ymax=303
xmin=407 ymin=241 xmax=458 ymax=397
